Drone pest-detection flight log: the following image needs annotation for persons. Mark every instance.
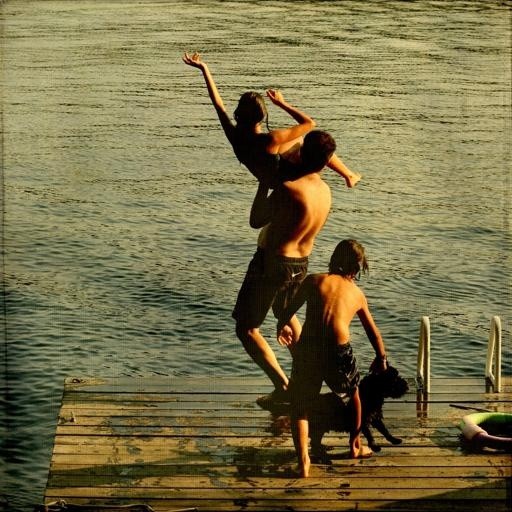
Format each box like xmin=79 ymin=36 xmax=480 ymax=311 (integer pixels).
xmin=231 ymin=128 xmax=334 ymax=407
xmin=181 ymin=50 xmax=363 ymax=191
xmin=274 ymin=237 xmax=389 ymax=478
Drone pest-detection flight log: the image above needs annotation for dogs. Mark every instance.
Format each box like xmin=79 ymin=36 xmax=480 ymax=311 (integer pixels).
xmin=308 ymin=361 xmax=409 ymax=465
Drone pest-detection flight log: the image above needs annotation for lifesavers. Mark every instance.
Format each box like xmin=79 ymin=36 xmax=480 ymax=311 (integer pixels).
xmin=460 ymin=412 xmax=512 ymax=454
